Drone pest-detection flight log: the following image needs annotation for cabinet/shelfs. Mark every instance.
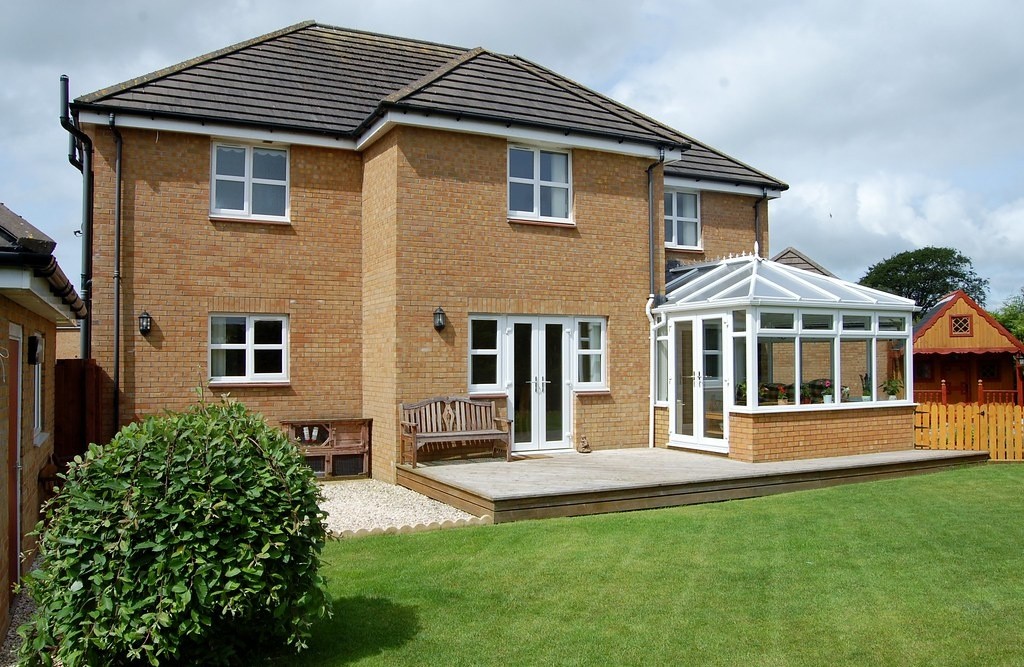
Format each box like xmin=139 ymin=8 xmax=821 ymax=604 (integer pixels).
xmin=276 ymin=417 xmax=372 ymax=480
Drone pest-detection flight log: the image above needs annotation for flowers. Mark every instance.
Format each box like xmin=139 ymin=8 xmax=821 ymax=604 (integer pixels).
xmin=820 ymin=379 xmax=833 ymax=395
xmin=738 ymin=383 xmax=770 ymax=398
xmin=776 ymin=384 xmax=788 ymax=400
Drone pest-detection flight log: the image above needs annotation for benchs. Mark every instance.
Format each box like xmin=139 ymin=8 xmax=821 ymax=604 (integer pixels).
xmin=398 ymin=396 xmax=514 ymax=470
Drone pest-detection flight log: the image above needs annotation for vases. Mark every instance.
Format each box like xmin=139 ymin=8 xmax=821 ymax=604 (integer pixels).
xmin=778 ymin=399 xmax=788 ymax=405
xmin=823 ymin=394 xmax=832 ymax=403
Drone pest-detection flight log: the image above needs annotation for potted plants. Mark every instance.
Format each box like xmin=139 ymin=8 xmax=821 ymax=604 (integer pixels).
xmin=878 ymin=372 xmax=904 ymax=400
xmin=858 ymin=373 xmax=872 ymax=402
xmin=801 ymin=382 xmax=812 ymax=404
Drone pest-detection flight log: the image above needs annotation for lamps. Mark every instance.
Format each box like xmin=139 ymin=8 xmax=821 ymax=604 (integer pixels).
xmin=137 ymin=310 xmax=152 ymax=338
xmin=27 ymin=335 xmax=46 ymax=366
xmin=432 ymin=306 xmax=445 ymax=333
